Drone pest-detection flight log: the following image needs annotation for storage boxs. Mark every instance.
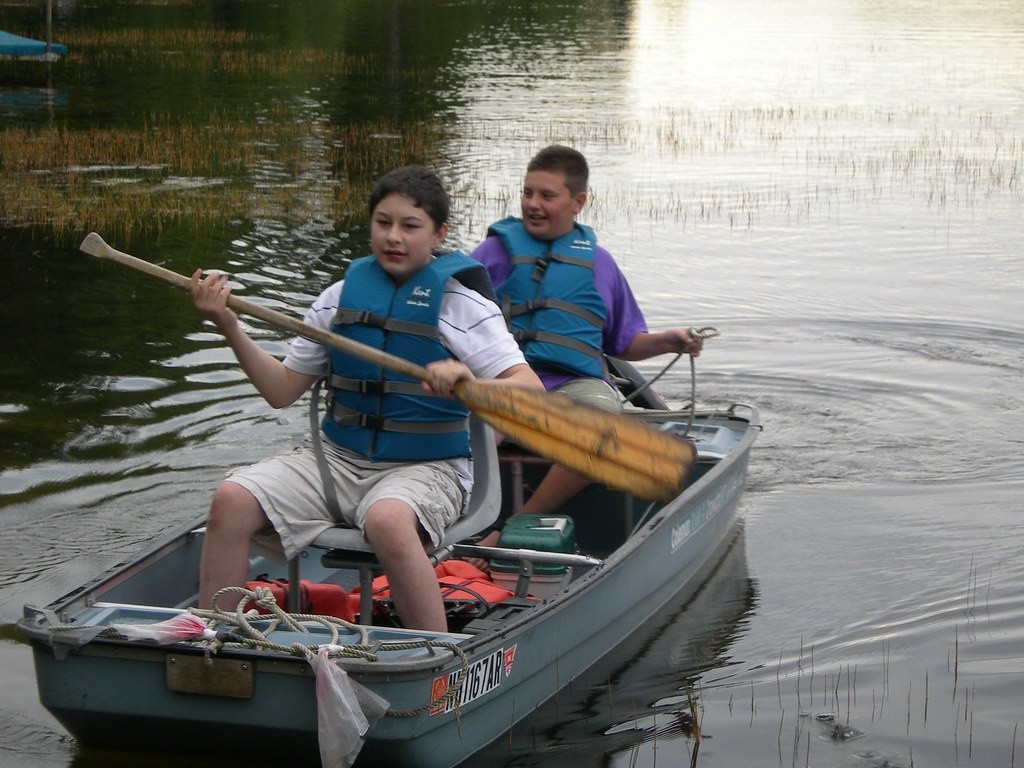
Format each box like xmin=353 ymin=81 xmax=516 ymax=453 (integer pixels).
xmin=489 ymin=512 xmax=579 ymax=600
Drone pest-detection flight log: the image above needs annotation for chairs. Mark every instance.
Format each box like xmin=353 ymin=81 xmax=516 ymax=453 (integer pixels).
xmin=288 ymin=400 xmax=635 ymax=626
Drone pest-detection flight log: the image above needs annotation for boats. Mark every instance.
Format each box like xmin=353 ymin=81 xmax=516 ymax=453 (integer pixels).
xmin=0 ymin=1 xmax=70 ymax=64
xmin=16 ymin=400 xmax=761 ymax=768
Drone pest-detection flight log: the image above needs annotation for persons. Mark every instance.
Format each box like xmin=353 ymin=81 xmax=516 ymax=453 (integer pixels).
xmin=460 ymin=144 xmax=703 ymax=572
xmin=191 ymin=164 xmax=549 ymax=634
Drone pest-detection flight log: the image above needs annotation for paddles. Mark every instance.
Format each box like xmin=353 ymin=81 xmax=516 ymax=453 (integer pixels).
xmin=79 ymin=230 xmax=696 ymax=504
